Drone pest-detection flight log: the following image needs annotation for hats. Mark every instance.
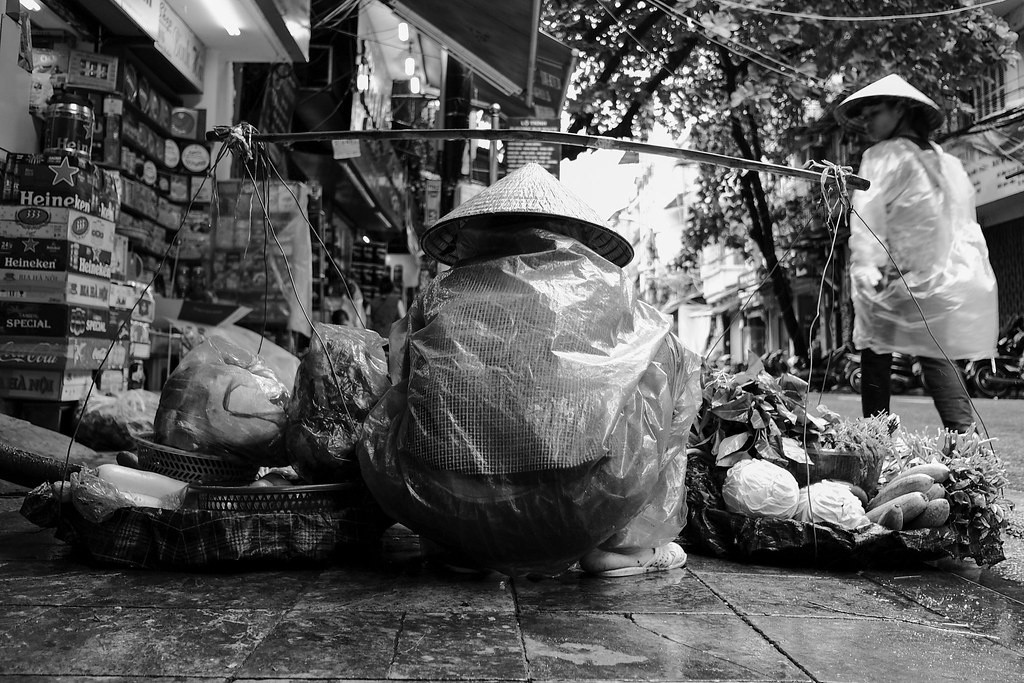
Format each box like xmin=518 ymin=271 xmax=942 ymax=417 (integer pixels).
xmin=833 ymin=73 xmax=944 ymax=135
xmin=421 ymin=163 xmax=634 ymax=269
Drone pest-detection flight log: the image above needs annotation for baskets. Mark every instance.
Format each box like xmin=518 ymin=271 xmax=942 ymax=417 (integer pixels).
xmin=796 ymin=448 xmax=884 ymax=495
xmin=185 ymin=477 xmax=353 ymax=514
xmin=127 ymin=430 xmax=261 ymax=484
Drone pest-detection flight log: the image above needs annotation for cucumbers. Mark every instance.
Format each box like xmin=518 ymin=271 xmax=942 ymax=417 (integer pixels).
xmin=861 ymin=461 xmax=950 ymax=530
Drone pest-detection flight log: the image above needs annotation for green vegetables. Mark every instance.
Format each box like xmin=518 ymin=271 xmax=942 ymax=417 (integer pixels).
xmin=686 ymin=350 xmax=830 ymax=467
xmin=944 ymin=465 xmax=1012 ymax=530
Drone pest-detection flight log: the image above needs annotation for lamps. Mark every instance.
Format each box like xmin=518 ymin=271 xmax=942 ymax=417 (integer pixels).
xmin=404 ymin=41 xmax=416 ymax=75
xmin=397 ymin=19 xmax=409 ymax=41
xmin=408 ymin=66 xmax=421 ymax=94
xmin=356 ymin=38 xmax=371 ymax=91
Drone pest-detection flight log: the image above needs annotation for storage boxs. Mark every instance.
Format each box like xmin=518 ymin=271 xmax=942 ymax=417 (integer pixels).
xmin=189 ymin=478 xmax=354 ymax=574
xmin=134 ymin=430 xmax=243 ymax=491
xmin=0 ymin=202 xmax=136 ymax=431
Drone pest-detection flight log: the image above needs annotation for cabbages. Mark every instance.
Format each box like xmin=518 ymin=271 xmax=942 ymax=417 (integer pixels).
xmin=797 ymin=478 xmax=870 ymax=531
xmin=721 ymin=458 xmax=800 ymax=519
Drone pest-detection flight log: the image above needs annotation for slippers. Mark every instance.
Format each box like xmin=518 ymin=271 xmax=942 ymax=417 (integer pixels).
xmin=584 ymin=542 xmax=687 ymax=577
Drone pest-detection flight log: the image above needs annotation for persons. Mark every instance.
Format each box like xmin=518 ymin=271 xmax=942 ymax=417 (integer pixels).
xmin=836 ymin=74 xmax=1000 ymax=465
xmin=367 ymin=159 xmax=702 ymax=577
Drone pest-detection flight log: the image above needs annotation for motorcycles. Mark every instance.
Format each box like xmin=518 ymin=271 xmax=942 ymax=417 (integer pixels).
xmin=734 ymin=313 xmax=1024 ymax=399
